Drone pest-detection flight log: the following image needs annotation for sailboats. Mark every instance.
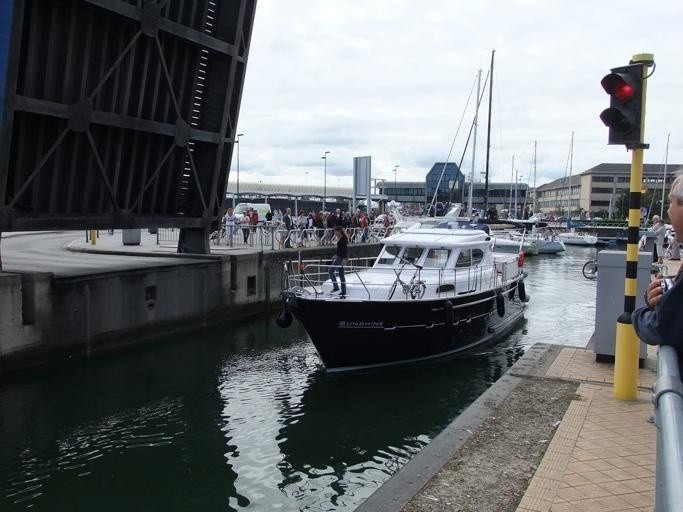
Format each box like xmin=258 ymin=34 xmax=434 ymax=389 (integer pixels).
xmin=639 ymin=133 xmax=671 ymax=237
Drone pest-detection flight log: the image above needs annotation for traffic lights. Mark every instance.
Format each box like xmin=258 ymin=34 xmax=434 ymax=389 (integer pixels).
xmin=600 ymin=63 xmax=644 ymax=145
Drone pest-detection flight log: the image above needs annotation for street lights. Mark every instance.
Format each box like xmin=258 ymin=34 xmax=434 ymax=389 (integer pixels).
xmin=236 ymin=133 xmax=243 ymax=207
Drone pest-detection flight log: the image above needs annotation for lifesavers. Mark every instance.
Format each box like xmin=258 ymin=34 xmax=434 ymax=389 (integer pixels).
xmin=519 ymin=251 xmax=524 ymax=266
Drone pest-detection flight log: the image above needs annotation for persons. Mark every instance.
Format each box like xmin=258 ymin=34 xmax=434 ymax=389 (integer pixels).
xmin=655 ymin=220 xmax=667 ymax=264
xmin=327 ymin=226 xmax=348 ymax=296
xmin=626 ymin=174 xmax=683 ymax=383
xmin=648 ymin=214 xmax=660 ymax=231
xmin=224 ymin=206 xmax=390 ymax=247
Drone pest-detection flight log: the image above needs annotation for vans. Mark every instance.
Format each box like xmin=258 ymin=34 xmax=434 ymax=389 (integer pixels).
xmin=233 ymin=203 xmax=272 ymax=224
